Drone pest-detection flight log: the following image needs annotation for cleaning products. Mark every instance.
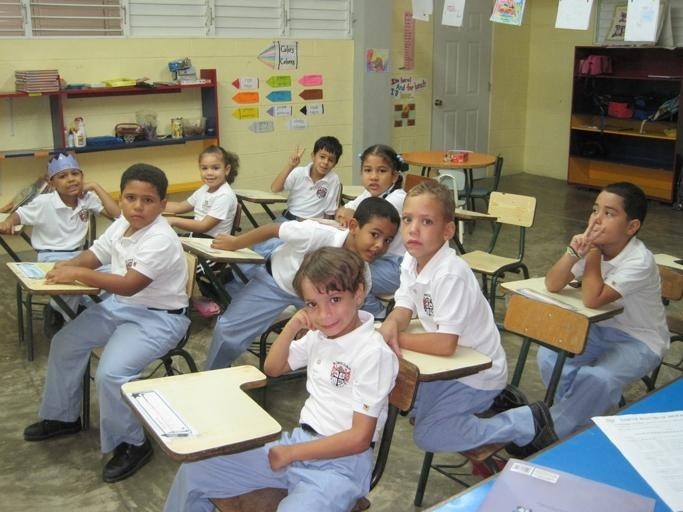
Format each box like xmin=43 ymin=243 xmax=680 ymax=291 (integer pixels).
xmin=72 ymin=117 xmax=87 ymax=148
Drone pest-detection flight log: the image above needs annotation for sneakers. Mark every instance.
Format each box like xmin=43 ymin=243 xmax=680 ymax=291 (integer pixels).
xmin=42 ymin=304 xmax=63 ymax=338
xmin=103 ymin=438 xmax=152 ymax=481
xmin=192 ymin=296 xmax=220 ymax=317
xmin=496 ymin=386 xmax=526 ymax=412
xmin=24 ymin=418 xmax=82 ymax=441
xmin=526 ymin=401 xmax=555 ymax=447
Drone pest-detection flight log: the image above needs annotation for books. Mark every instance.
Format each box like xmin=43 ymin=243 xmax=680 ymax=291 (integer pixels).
xmin=15 ymin=70 xmax=61 ymax=93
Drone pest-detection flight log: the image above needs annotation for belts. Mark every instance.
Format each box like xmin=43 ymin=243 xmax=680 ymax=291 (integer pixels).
xmin=265 ymin=257 xmax=272 ymax=273
xmin=154 ymin=309 xmax=188 ymax=315
xmin=282 ymin=209 xmax=299 ymax=220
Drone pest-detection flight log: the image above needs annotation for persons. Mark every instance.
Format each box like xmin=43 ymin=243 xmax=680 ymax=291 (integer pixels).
xmin=161 ymin=143 xmax=239 ymax=315
xmin=335 ymin=143 xmax=407 ymax=323
xmin=22 ymin=162 xmax=189 ymax=484
xmin=0 ymin=153 xmax=121 ymax=339
xmin=206 ymin=197 xmax=400 ymax=372
xmin=379 ymin=181 xmax=559 ymax=461
xmin=162 ymin=245 xmax=399 ymax=512
xmin=216 ymin=137 xmax=342 ymax=298
xmin=536 ymin=180 xmax=670 ymax=439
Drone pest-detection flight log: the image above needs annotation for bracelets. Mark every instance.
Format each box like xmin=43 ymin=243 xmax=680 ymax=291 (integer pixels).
xmin=567 ymin=245 xmax=582 ymax=262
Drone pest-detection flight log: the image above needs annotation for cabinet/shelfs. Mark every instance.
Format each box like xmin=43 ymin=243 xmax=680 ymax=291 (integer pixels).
xmin=567 ymin=45 xmax=682 ymax=203
xmin=0 ymin=68 xmax=220 ymax=212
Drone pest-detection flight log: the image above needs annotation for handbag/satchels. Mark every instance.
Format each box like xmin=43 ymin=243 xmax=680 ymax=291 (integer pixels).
xmin=653 ymin=95 xmax=679 ymax=121
xmin=582 ymin=56 xmax=611 ymax=75
